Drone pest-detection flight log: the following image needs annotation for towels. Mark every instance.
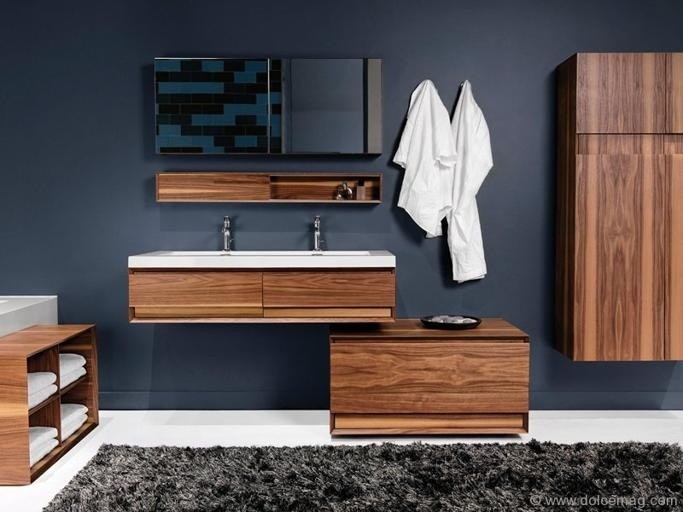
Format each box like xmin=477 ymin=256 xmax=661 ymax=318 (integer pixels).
xmin=27 ymin=353 xmax=89 ymax=468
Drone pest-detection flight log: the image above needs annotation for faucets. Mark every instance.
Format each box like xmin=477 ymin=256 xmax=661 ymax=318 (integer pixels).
xmin=314 ymin=215 xmax=324 ymax=251
xmin=221 ymin=215 xmax=232 ymax=250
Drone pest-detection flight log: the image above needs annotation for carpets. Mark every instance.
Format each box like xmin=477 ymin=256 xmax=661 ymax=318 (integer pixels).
xmin=39 ymin=445 xmax=682 ymax=511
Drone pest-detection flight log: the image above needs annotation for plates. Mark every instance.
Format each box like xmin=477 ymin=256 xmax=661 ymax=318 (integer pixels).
xmin=419 ymin=314 xmax=480 ymax=330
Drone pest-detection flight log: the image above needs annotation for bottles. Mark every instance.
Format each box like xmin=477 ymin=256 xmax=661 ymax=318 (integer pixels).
xmin=335 ymin=180 xmax=368 ymax=201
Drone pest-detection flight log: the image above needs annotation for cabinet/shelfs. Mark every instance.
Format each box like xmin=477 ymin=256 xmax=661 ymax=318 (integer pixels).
xmin=326 ymin=315 xmax=530 ymax=436
xmin=1 ymin=324 xmax=99 ymax=486
xmin=152 ymin=170 xmax=386 ymax=205
xmin=557 ymin=51 xmax=681 ymax=366
xmin=126 ymin=270 xmax=397 ymax=326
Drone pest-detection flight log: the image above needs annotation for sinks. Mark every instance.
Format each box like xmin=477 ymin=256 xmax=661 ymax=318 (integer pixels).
xmin=127 ymin=249 xmax=397 ymax=272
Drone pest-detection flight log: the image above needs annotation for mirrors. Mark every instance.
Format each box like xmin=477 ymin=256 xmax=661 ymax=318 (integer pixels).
xmin=153 ymin=55 xmax=382 ymax=158
